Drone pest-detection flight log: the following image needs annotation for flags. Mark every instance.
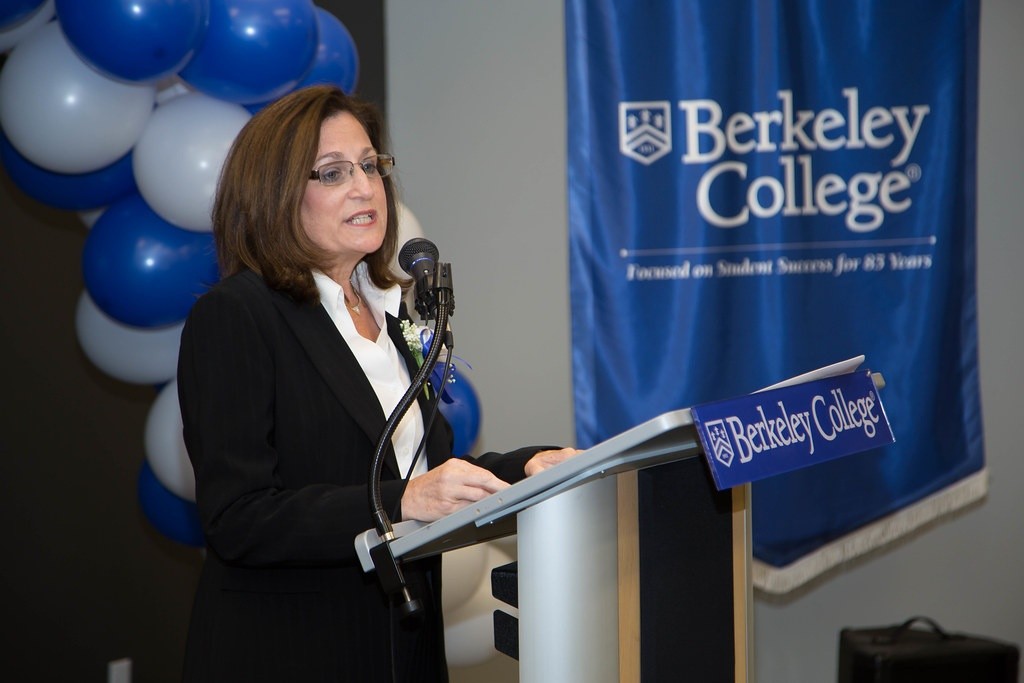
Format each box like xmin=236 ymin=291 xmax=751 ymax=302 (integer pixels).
xmin=559 ymin=0 xmax=988 ymax=601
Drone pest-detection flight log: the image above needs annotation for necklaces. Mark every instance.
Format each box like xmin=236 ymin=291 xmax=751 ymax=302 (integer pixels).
xmin=344 ymin=278 xmax=363 ymax=318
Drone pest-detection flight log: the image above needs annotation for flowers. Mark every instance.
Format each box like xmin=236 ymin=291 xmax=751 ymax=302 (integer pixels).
xmin=401 ymin=320 xmax=456 ymax=401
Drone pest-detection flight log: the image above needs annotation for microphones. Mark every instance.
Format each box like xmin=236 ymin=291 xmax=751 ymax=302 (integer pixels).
xmin=398 ymin=238 xmax=454 ymax=349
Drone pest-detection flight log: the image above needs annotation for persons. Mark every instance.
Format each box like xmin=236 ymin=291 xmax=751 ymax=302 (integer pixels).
xmin=173 ymin=86 xmax=591 ymax=682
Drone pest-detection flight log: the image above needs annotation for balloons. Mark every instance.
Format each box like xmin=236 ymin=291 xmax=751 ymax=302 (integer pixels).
xmin=2 ymin=0 xmax=486 ymax=544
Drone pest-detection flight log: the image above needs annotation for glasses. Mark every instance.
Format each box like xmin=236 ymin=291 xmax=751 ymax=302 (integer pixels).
xmin=306 ymin=153 xmax=395 ymax=186
